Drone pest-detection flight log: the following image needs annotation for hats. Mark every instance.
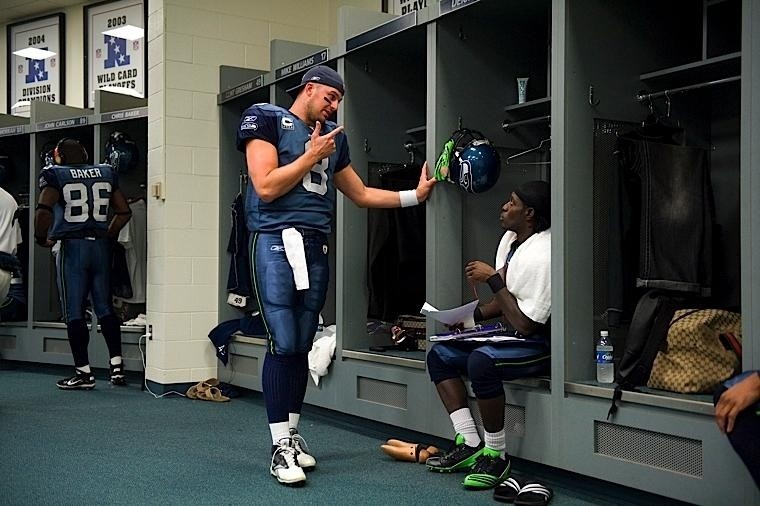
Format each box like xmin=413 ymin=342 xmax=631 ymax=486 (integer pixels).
xmin=286 ymin=66 xmax=344 ymax=100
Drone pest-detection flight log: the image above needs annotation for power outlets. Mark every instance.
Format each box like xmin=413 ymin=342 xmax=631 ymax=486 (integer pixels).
xmin=147 ymin=325 xmax=152 ymax=339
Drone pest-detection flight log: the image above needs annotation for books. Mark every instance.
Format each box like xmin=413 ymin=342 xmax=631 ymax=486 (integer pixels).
xmin=427 ymin=321 xmax=509 ymax=343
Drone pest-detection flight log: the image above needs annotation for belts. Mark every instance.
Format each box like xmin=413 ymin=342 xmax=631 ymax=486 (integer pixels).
xmin=299 ymin=229 xmax=326 ymax=236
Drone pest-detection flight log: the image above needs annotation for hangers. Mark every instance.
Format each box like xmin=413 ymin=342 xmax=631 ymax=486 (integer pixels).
xmin=654 ymin=88 xmax=677 ymax=121
xmin=507 ymin=115 xmax=560 ymax=165
xmin=615 ymin=89 xmax=719 ymax=151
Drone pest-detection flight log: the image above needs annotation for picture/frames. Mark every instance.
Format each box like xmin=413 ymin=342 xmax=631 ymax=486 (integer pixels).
xmin=6 ymin=11 xmax=65 ymax=116
xmin=380 ymin=0 xmax=428 ymax=17
xmin=82 ymin=0 xmax=150 ymax=107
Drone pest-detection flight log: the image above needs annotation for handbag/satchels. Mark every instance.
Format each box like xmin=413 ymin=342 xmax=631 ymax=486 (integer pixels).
xmin=646 ymin=309 xmax=742 ymax=394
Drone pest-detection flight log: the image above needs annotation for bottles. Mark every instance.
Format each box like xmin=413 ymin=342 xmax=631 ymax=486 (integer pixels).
xmin=596 ymin=331 xmax=614 ymax=383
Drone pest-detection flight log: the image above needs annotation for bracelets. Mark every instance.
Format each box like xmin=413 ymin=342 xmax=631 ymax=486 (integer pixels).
xmin=485 ymin=272 xmax=506 ymax=294
xmin=474 ymin=308 xmax=484 ymax=323
xmin=34 ymin=233 xmax=47 ymax=245
xmin=399 ymin=188 xmax=419 ymax=208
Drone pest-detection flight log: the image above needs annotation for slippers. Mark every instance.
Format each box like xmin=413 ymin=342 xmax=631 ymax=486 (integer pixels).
xmin=493 ymin=476 xmax=524 ymax=502
xmin=514 ymin=481 xmax=553 ymax=506
xmin=197 ymin=387 xmax=231 ymax=402
xmin=186 ymin=378 xmax=220 ymax=399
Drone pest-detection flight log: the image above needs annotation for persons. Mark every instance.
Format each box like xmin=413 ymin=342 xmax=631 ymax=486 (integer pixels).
xmin=34 ymin=138 xmax=133 ymax=390
xmin=711 ymin=370 xmax=760 ymax=490
xmin=1 ymin=187 xmax=24 ymax=308
xmin=235 ymin=64 xmax=440 ymax=488
xmin=424 ymin=180 xmax=551 ymax=492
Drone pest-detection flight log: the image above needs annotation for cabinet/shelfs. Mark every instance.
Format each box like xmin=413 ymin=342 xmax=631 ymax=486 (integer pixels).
xmin=32 ymin=100 xmax=98 ymax=370
xmin=431 ymin=0 xmax=561 ymax=471
xmin=342 ymin=0 xmax=430 ymax=442
xmin=98 ymin=92 xmax=150 ymax=378
xmin=1 ymin=110 xmax=32 ymax=369
xmin=562 ymin=0 xmax=760 ymax=506
xmin=272 ymin=34 xmax=343 ymax=425
xmin=218 ymin=61 xmax=271 ymax=400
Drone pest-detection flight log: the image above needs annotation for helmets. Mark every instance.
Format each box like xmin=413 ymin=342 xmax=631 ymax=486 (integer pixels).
xmin=434 ymin=138 xmax=501 ymax=193
xmin=105 ymin=133 xmax=136 ymax=172
xmin=54 ymin=139 xmax=89 ymax=165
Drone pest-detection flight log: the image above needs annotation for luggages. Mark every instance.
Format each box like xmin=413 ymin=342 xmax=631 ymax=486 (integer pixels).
xmin=397 ymin=315 xmax=426 ymax=351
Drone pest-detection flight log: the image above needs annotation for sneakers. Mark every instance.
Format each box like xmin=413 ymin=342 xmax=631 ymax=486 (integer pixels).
xmin=426 ymin=433 xmax=485 ymax=472
xmin=109 ymin=359 xmax=127 ymax=386
xmin=56 ymin=368 xmax=96 ymax=390
xmin=464 ymin=447 xmax=511 ymax=488
xmin=270 ymin=438 xmax=306 ymax=487
xmin=290 ymin=429 xmax=316 ymax=472
